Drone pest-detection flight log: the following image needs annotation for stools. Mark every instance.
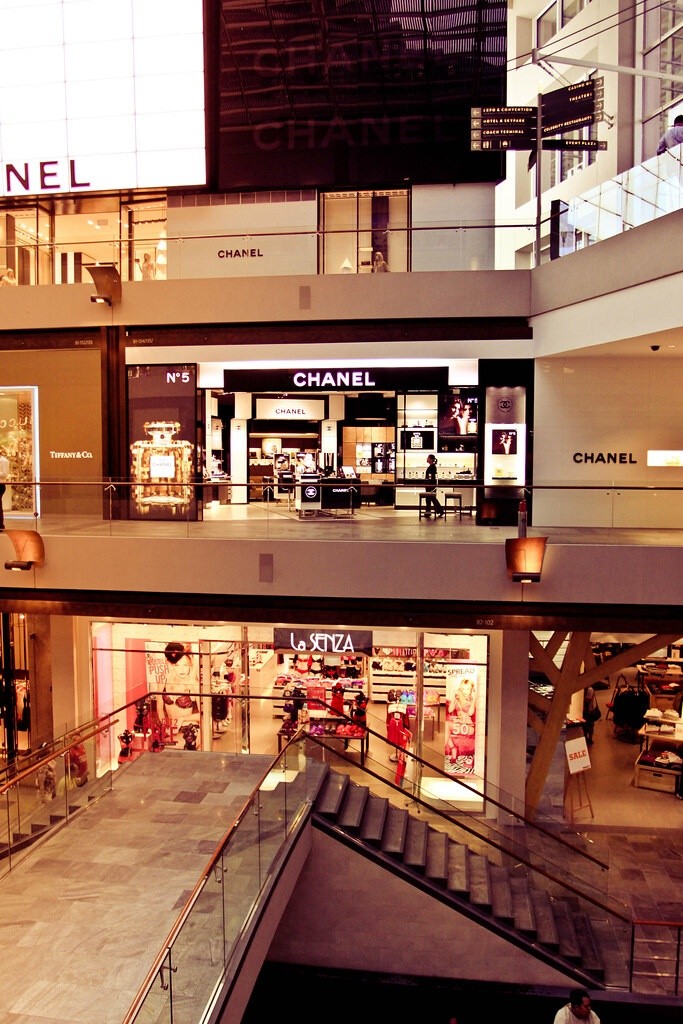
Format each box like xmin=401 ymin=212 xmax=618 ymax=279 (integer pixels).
xmin=444 ymin=492 xmax=461 ymax=521
xmin=418 ymin=492 xmax=436 ymax=521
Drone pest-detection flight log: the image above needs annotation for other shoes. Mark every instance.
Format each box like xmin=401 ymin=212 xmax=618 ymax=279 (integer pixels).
xmin=585 ymin=738 xmax=593 ymax=745
xmin=422 ymin=514 xmax=431 ymax=518
xmin=438 ymin=512 xmax=444 ymax=517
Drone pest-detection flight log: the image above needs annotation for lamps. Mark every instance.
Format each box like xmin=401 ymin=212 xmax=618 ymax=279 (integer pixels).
xmin=85 ymin=266 xmax=121 ymax=306
xmin=1 ymin=528 xmax=45 ymax=571
xmin=504 ymin=537 xmax=547 ymax=583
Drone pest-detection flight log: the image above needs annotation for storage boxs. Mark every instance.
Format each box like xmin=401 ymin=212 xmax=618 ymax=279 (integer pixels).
xmin=633 ymin=749 xmax=680 ymax=793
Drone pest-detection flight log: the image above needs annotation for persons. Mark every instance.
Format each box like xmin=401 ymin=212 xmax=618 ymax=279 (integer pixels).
xmin=374 ymin=252 xmax=390 ymax=273
xmin=441 ymin=397 xmax=474 ymax=435
xmin=442 ymin=679 xmax=475 ymax=763
xmin=493 ymin=431 xmax=516 ymax=454
xmin=657 ymin=115 xmax=683 ymax=155
xmin=35 ymin=732 xmax=89 ymax=805
xmin=139 ymin=253 xmax=157 ymax=281
xmin=554 ymin=988 xmax=600 ymax=1024
xmin=1 ymin=268 xmax=17 ymax=286
xmin=424 ymin=454 xmax=444 ymax=518
xmin=157 ymin=649 xmax=200 ymax=728
xmin=583 ymin=686 xmax=597 ymax=746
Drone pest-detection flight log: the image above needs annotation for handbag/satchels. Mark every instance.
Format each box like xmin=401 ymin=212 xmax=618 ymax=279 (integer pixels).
xmin=588 ymin=707 xmax=601 ymax=722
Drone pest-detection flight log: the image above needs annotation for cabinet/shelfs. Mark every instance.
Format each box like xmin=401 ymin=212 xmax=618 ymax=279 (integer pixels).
xmin=217 ymin=481 xmax=232 ymax=505
xmin=643 ymin=682 xmax=682 ymax=710
xmin=636 ymin=664 xmax=682 ymax=711
xmin=209 ymin=640 xmax=469 ymax=778
xmin=638 ymin=711 xmax=682 ymax=800
